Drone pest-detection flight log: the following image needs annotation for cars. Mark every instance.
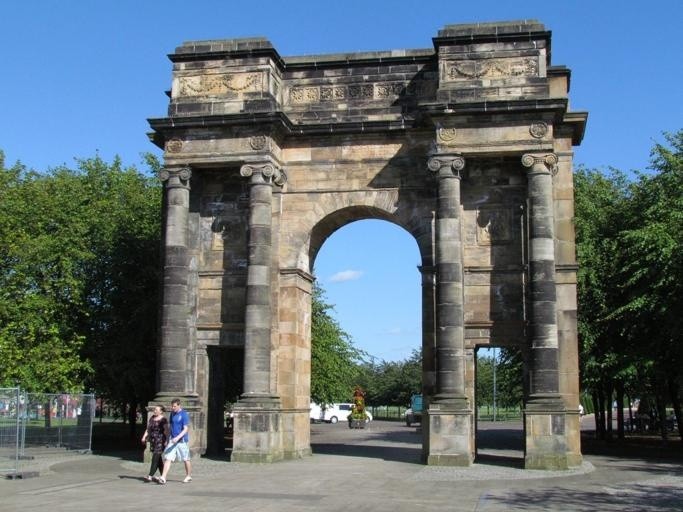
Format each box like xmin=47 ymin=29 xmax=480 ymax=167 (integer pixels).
xmin=309 ymin=402 xmax=322 ymax=424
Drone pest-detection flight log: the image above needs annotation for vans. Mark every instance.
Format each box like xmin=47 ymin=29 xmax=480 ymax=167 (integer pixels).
xmin=320 ymin=402 xmax=373 ymax=425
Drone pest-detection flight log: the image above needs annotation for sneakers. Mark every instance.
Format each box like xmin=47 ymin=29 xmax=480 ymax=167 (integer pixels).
xmin=144 ymin=474 xmax=169 ymax=484
xmin=183 ymin=475 xmax=194 ymax=483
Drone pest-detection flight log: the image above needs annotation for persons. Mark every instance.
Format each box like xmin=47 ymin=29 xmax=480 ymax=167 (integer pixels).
xmin=152 ymin=398 xmax=194 ymax=483
xmin=140 ymin=405 xmax=169 ymax=483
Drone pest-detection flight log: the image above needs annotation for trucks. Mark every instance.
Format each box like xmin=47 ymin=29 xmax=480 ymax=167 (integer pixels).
xmin=403 ymin=395 xmax=422 ymax=427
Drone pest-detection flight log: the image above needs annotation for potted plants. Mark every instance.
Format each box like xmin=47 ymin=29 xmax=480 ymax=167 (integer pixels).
xmin=347 ymin=386 xmax=366 ymax=429
